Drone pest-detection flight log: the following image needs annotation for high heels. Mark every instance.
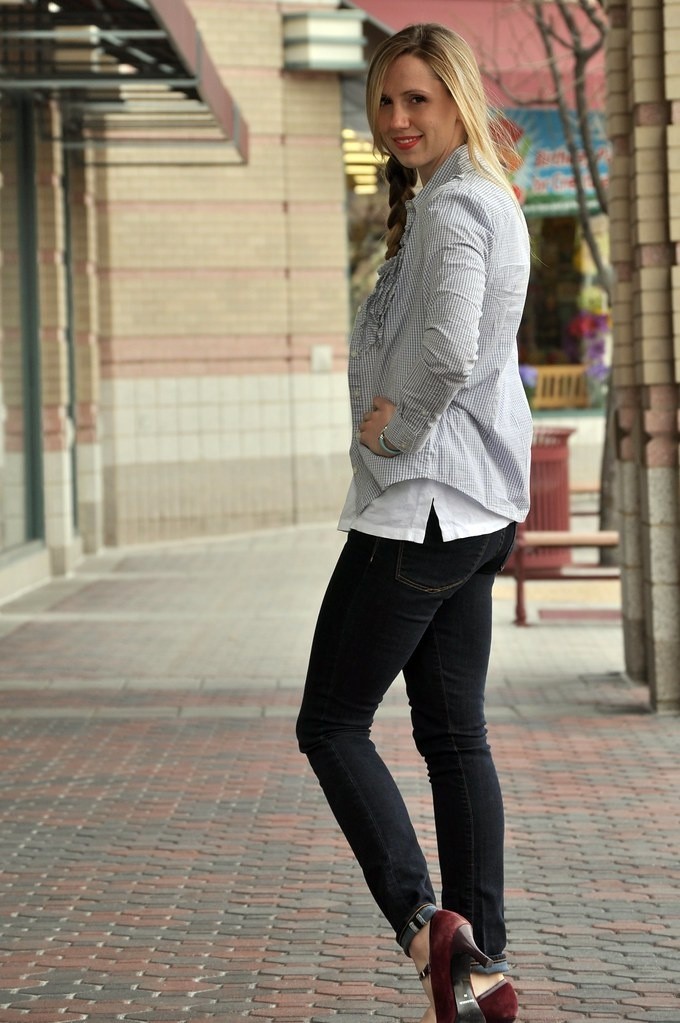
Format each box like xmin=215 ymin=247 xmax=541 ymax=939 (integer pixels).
xmin=418 ymin=910 xmax=493 ymax=1023
xmin=476 ymin=979 xmax=518 ymax=1023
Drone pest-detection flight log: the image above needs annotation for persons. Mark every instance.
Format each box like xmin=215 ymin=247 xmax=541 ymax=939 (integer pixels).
xmin=295 ymin=24 xmax=533 ymax=1022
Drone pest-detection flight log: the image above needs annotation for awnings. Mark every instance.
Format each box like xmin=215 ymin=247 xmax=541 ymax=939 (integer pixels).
xmin=1 ymin=0 xmax=250 ymax=166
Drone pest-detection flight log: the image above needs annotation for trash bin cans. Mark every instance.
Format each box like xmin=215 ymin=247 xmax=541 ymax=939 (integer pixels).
xmin=499 ymin=426 xmax=578 ymax=577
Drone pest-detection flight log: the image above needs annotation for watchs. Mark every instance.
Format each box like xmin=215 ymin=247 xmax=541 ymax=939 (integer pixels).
xmin=377 ymin=424 xmax=402 ymax=455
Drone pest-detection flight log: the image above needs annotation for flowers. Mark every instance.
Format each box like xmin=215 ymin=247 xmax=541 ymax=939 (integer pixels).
xmin=518 ymin=366 xmax=537 ymax=405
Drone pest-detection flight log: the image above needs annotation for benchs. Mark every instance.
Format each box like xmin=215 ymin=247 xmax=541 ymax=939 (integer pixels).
xmin=511 ymin=531 xmax=620 ymax=626
